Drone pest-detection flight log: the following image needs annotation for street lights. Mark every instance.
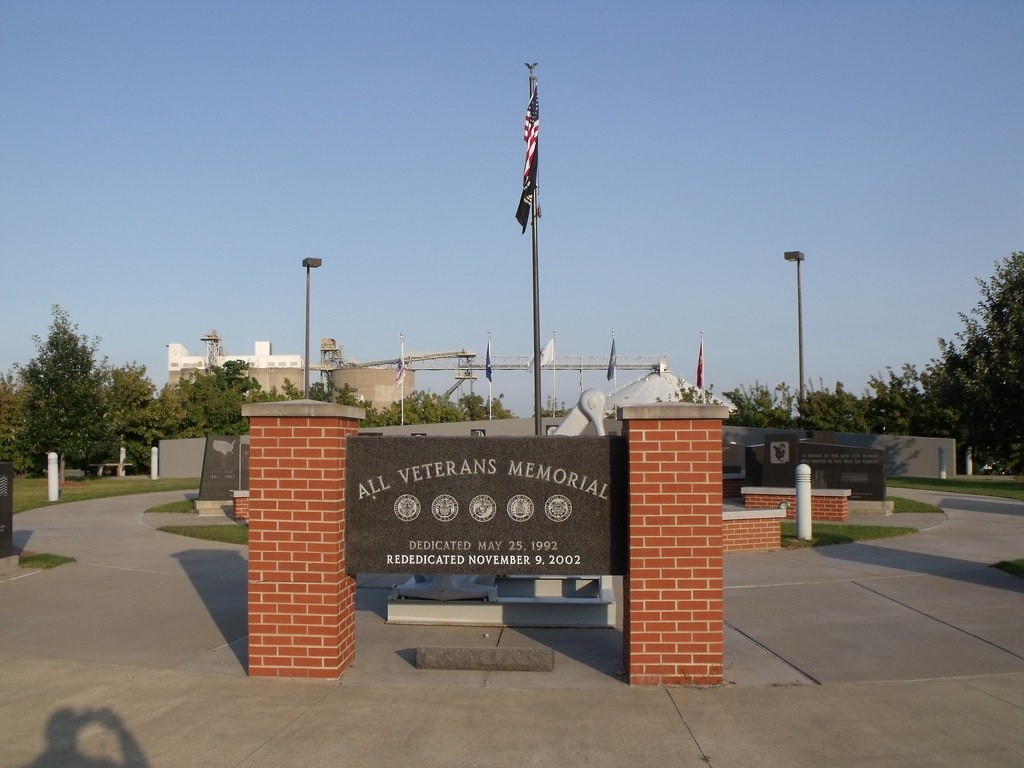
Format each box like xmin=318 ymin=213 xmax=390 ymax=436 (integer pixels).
xmin=303 ymin=258 xmax=322 ymax=399
xmin=785 ymin=250 xmax=805 ymax=419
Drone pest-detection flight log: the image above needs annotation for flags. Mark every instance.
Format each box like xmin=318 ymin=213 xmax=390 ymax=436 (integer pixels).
xmin=395 ymin=358 xmax=406 ymax=387
xmin=607 ymin=339 xmax=617 ymax=381
xmin=527 ymin=338 xmax=553 ymax=374
xmin=516 ymin=87 xmax=540 ymax=236
xmin=485 ymin=342 xmax=492 ymax=382
xmin=697 ymin=342 xmax=703 ymax=388
